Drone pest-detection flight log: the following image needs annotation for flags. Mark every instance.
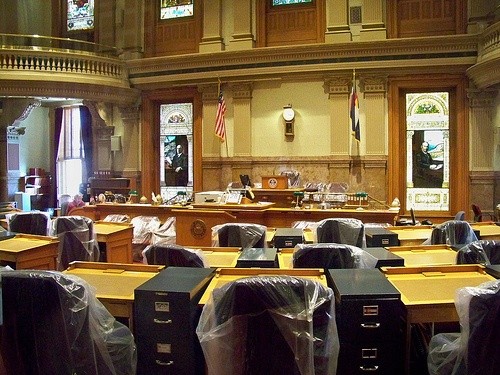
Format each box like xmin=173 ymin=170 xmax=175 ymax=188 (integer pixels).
xmin=350 ymin=86 xmax=360 ymax=142
xmin=214 ymin=91 xmax=226 ymax=141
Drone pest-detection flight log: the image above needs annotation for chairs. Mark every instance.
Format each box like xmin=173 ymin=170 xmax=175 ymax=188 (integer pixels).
xmin=453 ymin=211 xmax=466 ymax=222
xmin=292 ymin=243 xmax=378 ymax=270
xmin=456 ymin=237 xmax=500 ymax=266
xmin=196 ymin=274 xmax=340 ymax=375
xmin=429 ymin=219 xmax=479 ymax=246
xmin=470 ymin=203 xmax=499 ymax=222
xmin=5 ymin=211 xmax=52 ymax=237
xmin=426 ymin=278 xmax=500 ymax=375
xmin=1 ymin=265 xmax=138 ymax=375
xmin=211 ymin=223 xmax=267 ymax=249
xmin=51 ymin=216 xmax=101 ymax=270
xmin=142 ymin=241 xmax=205 ymax=269
xmin=316 ymin=217 xmax=366 ymax=249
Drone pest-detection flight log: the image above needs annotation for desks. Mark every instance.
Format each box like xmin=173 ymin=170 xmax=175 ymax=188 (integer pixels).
xmin=0 ymin=188 xmax=500 ymax=375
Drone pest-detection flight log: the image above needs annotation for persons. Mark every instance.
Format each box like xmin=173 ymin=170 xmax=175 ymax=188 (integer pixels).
xmin=172 ymin=145 xmax=188 ymax=195
xmin=66 ymin=192 xmax=85 ymax=214
xmin=416 ymin=142 xmax=436 ymax=180
xmin=89 ymin=193 xmax=105 ymax=205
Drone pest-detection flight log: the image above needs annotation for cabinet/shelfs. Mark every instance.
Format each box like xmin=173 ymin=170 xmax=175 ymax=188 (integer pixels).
xmin=91 ymin=178 xmax=131 ymax=198
xmin=24 ymin=176 xmax=50 ymax=193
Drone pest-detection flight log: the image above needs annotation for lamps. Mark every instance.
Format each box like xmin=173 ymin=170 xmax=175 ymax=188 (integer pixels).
xmin=294 ymin=192 xmax=304 ymax=209
xmin=282 ymin=104 xmax=295 ymax=137
xmin=355 ymin=191 xmax=366 ymax=211
xmin=126 ymin=190 xmax=139 ymax=203
xmin=163 ymin=192 xmax=187 ymax=204
